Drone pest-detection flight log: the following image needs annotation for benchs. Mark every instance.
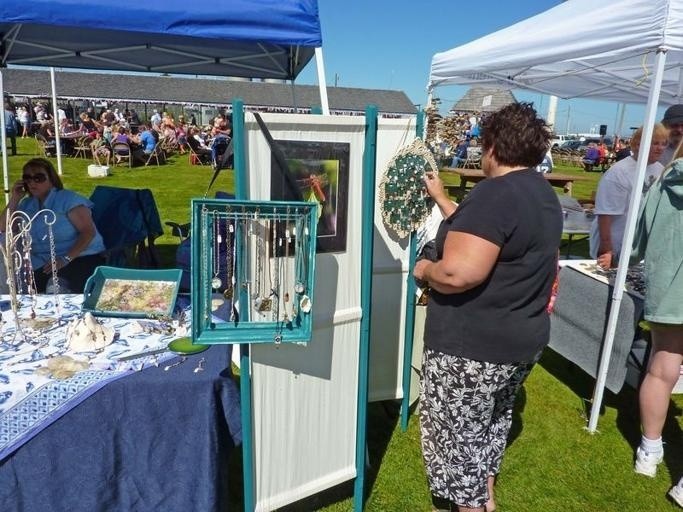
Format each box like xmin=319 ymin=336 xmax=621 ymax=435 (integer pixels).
xmin=442 ymin=183 xmax=473 ymax=198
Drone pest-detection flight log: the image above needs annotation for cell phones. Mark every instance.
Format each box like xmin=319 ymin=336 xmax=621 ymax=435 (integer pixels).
xmin=22 ymin=182 xmax=27 ymax=193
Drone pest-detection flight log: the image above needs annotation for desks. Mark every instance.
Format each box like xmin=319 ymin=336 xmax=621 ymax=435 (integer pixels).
xmin=0 ymin=294 xmax=241 ymax=511
xmin=553 ymin=206 xmax=594 ymax=260
xmin=547 ymin=261 xmax=650 ymax=401
xmin=440 ymin=166 xmax=592 ymax=205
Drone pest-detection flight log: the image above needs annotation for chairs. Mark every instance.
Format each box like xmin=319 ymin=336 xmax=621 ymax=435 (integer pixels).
xmin=555 ymin=143 xmax=617 ymax=173
xmin=13 ymin=108 xmax=233 ymax=169
xmin=87 ymin=182 xmax=164 ymax=270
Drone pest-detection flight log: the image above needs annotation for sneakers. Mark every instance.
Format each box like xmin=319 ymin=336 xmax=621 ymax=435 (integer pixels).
xmin=668 ymin=477 xmax=683 ymax=508
xmin=634 ymin=442 xmax=667 ymax=477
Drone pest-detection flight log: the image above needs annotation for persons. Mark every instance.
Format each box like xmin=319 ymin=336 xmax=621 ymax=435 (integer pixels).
xmin=0 ymin=158 xmax=113 ymax=294
xmin=3 ymin=99 xmax=233 ymax=166
xmin=579 ymin=139 xmax=634 ymax=171
xmin=657 ymin=104 xmax=683 ymax=167
xmin=589 ymin=122 xmax=670 ymax=395
xmin=450 ymin=139 xmax=472 ymax=168
xmin=412 ymin=101 xmax=563 ymax=512
xmin=597 ymin=157 xmax=683 ymax=508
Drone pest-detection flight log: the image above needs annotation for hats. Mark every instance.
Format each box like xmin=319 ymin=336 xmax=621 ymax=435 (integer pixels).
xmin=664 ymin=104 xmax=683 ymax=126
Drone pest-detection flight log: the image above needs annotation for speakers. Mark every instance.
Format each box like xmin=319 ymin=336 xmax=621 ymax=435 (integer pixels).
xmin=600 ymin=125 xmax=607 ymax=135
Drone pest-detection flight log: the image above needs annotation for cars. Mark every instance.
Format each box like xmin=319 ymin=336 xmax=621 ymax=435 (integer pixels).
xmin=551 ymin=133 xmax=614 ymax=153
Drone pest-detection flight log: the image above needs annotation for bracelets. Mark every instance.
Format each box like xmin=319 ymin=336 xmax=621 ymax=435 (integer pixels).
xmin=63 ymin=254 xmax=72 ymax=262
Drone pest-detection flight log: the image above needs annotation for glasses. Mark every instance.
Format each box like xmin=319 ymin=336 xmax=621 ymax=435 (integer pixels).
xmin=23 ymin=173 xmax=46 ymax=183
